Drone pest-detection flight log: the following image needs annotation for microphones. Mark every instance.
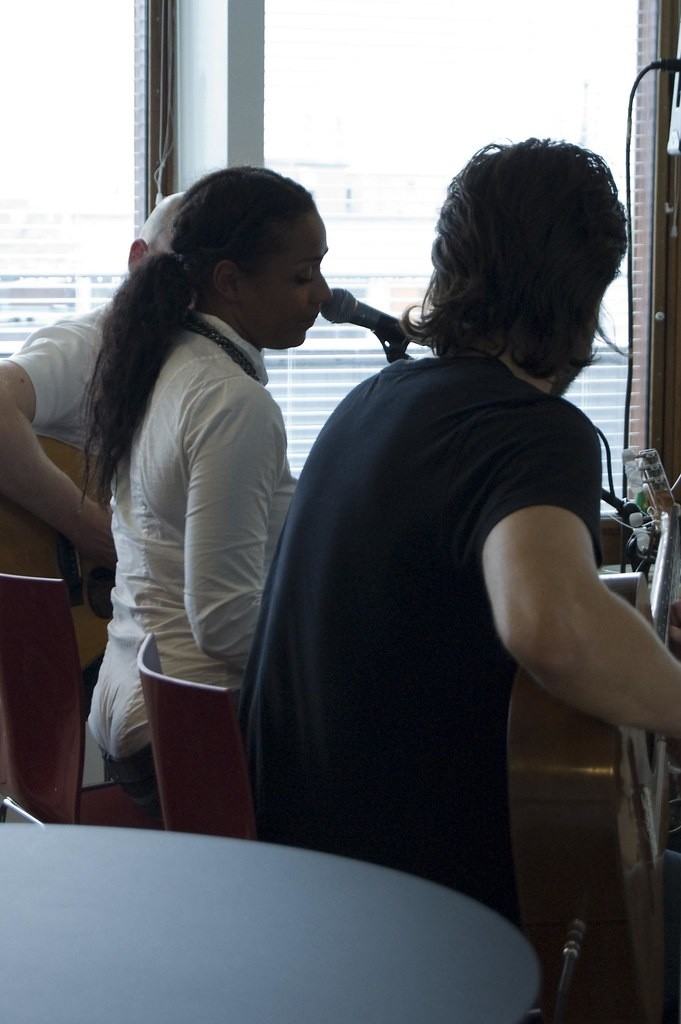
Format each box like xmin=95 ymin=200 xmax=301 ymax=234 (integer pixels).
xmin=320 ymin=286 xmax=425 ymax=345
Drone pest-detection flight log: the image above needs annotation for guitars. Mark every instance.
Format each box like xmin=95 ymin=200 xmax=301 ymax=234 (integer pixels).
xmin=504 ymin=446 xmax=681 ymax=1024
xmin=0 ymin=430 xmax=107 ymax=671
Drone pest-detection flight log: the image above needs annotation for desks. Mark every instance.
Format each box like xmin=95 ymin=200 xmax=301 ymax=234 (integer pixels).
xmin=0 ymin=821 xmax=540 ymax=1024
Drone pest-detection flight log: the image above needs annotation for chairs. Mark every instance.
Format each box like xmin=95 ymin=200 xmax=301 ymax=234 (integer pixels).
xmin=133 ymin=633 xmax=257 ymax=841
xmin=0 ymin=573 xmax=154 ymax=829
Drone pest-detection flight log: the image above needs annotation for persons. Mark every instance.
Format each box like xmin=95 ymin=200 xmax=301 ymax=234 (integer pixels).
xmin=0 ymin=189 xmax=187 ymax=570
xmin=88 ymin=169 xmax=327 ymax=825
xmin=236 ymin=140 xmax=681 ymax=1024
